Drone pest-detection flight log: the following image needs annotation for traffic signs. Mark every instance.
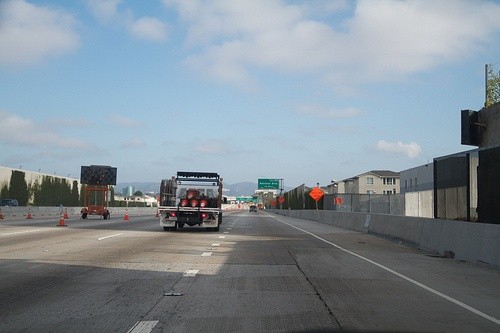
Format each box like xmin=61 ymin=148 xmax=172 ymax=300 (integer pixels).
xmin=257 ymin=178 xmax=279 ymax=189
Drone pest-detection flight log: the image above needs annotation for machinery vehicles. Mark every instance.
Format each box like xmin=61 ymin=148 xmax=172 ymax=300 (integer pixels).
xmin=157 ymin=171 xmax=227 ymax=231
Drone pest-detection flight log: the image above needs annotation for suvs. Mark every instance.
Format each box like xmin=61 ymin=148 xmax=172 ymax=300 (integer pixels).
xmin=1 ymin=198 xmax=18 ymax=207
xmin=249 ymin=205 xmax=257 ymax=212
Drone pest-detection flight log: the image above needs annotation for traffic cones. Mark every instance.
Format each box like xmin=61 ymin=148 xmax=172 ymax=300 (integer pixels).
xmin=0 ymin=208 xmax=5 ymax=219
xmin=56 ymin=207 xmax=68 ymax=226
xmin=26 ymin=207 xmax=34 ymax=219
xmin=64 ymin=208 xmax=69 ymax=219
xmin=123 ymin=208 xmax=130 ymax=220
